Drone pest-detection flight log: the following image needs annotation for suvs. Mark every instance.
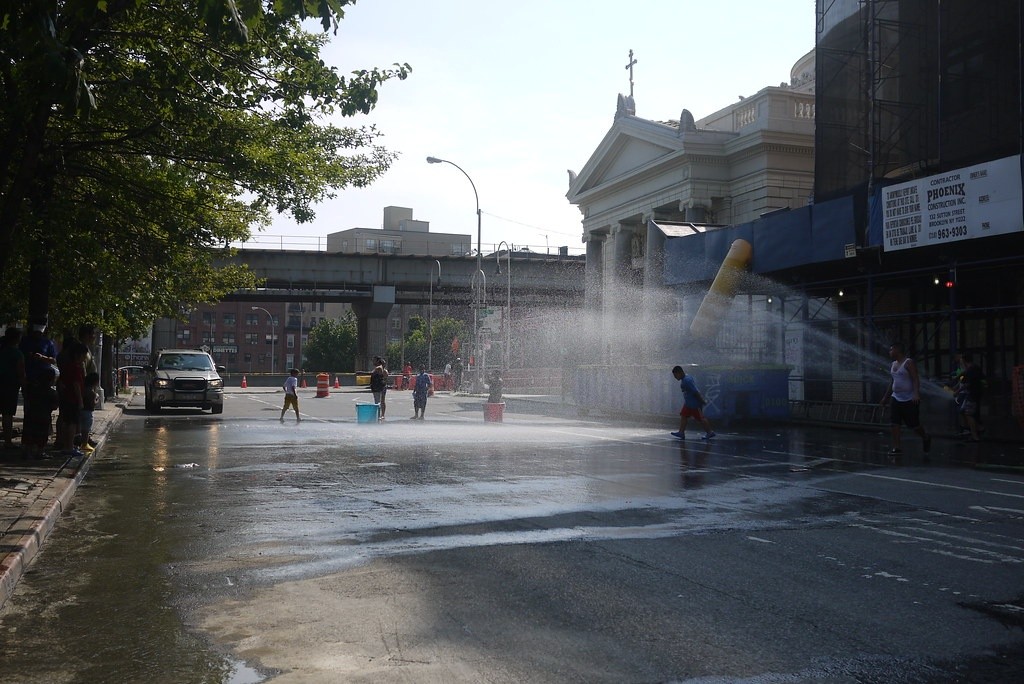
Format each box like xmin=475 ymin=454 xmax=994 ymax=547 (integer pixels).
xmin=142 ymin=347 xmax=227 ymax=414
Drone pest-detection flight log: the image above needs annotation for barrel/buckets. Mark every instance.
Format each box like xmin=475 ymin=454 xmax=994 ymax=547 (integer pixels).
xmin=482 ymin=403 xmax=505 ymax=423
xmin=355 ymin=402 xmax=379 ymax=424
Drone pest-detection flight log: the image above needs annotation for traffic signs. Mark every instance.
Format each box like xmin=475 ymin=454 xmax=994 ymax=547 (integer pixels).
xmin=467 ymin=302 xmax=488 ymax=310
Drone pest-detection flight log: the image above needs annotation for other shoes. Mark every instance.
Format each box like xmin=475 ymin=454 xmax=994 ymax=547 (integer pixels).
xmin=378 ymin=415 xmax=385 ymax=420
xmin=887 ymin=448 xmax=904 ymax=454
xmin=297 ymin=418 xmax=303 ymax=422
xmin=671 ymin=431 xmax=686 ymax=439
xmin=419 ymin=415 xmax=424 ymax=420
xmin=410 ymin=416 xmax=418 ymax=419
xmin=702 ymin=431 xmax=716 ymax=439
xmin=74 ymin=434 xmax=97 ymax=446
xmin=27 ymin=444 xmax=52 ymax=460
xmin=977 ymin=427 xmax=985 ymax=433
xmin=923 ymin=434 xmax=931 ymax=453
xmin=279 ymin=418 xmax=283 ymax=422
xmin=963 ymin=436 xmax=980 ymax=443
xmin=79 ymin=443 xmax=95 ymax=452
xmin=953 ymin=429 xmax=970 ymax=437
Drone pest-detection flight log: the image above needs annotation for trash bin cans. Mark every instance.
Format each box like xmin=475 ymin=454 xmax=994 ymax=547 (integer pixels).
xmin=356 ymin=371 xmax=372 ymax=386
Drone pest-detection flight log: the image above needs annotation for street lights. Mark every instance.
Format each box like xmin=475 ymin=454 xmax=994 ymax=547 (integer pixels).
xmin=494 ymin=241 xmax=511 ymax=372
xmin=426 ymin=156 xmax=482 ymax=395
xmin=251 ymin=306 xmax=275 ymax=373
xmin=428 ymin=259 xmax=443 ymax=374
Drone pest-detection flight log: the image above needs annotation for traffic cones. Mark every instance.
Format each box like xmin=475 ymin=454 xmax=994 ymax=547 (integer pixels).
xmin=333 ymin=377 xmax=339 ymax=388
xmin=240 ymin=374 xmax=248 ymax=388
xmin=302 ymin=379 xmax=307 ymax=388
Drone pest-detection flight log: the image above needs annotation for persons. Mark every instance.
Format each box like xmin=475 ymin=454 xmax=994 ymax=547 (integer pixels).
xmin=370 ymin=355 xmax=388 ymax=421
xmin=444 ymin=357 xmax=463 ymax=393
xmin=0 ymin=318 xmax=99 ymax=459
xmin=401 ymin=361 xmax=412 ymax=391
xmin=879 ymin=342 xmax=931 ymax=455
xmin=946 ymin=351 xmax=984 ymax=442
xmin=487 ymin=369 xmax=503 ymax=404
xmin=671 ymin=366 xmax=716 ymax=440
xmin=410 ymin=363 xmax=431 ymax=421
xmin=280 ymin=368 xmax=302 ymax=424
